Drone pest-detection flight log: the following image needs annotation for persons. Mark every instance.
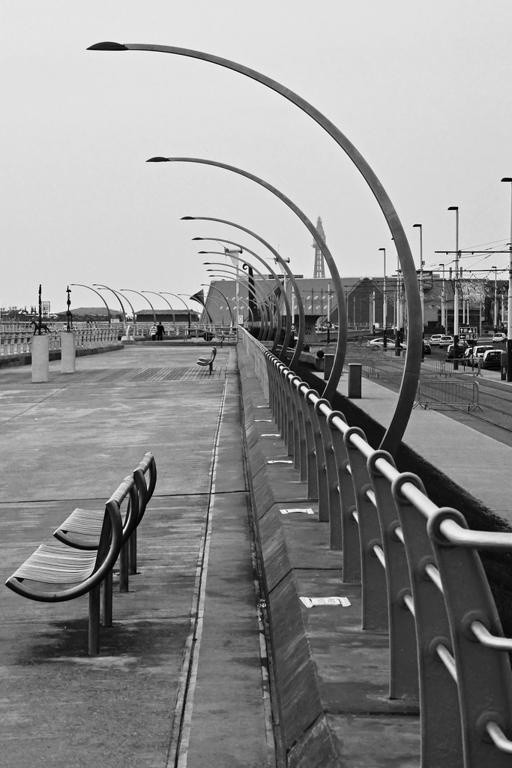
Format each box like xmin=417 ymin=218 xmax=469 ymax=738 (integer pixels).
xmin=156 ymin=321 xmax=165 ymax=341
xmin=149 ymin=323 xmax=158 ymax=341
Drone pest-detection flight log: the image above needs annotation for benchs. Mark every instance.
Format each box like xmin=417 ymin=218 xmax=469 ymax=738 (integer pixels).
xmin=6 ymin=452 xmax=158 ymax=656
xmin=197 ymin=345 xmax=218 ymax=375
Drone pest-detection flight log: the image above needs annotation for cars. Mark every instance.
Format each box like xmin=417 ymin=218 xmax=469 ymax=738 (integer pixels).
xmin=364 ymin=333 xmax=512 ymax=370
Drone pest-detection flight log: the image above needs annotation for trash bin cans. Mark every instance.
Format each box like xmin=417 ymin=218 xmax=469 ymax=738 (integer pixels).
xmin=324 ymin=354 xmax=335 ymax=380
xmin=454 ymin=360 xmax=458 ymax=369
xmin=395 ymin=347 xmax=401 ymax=356
xmin=348 ymin=362 xmax=362 ymax=399
